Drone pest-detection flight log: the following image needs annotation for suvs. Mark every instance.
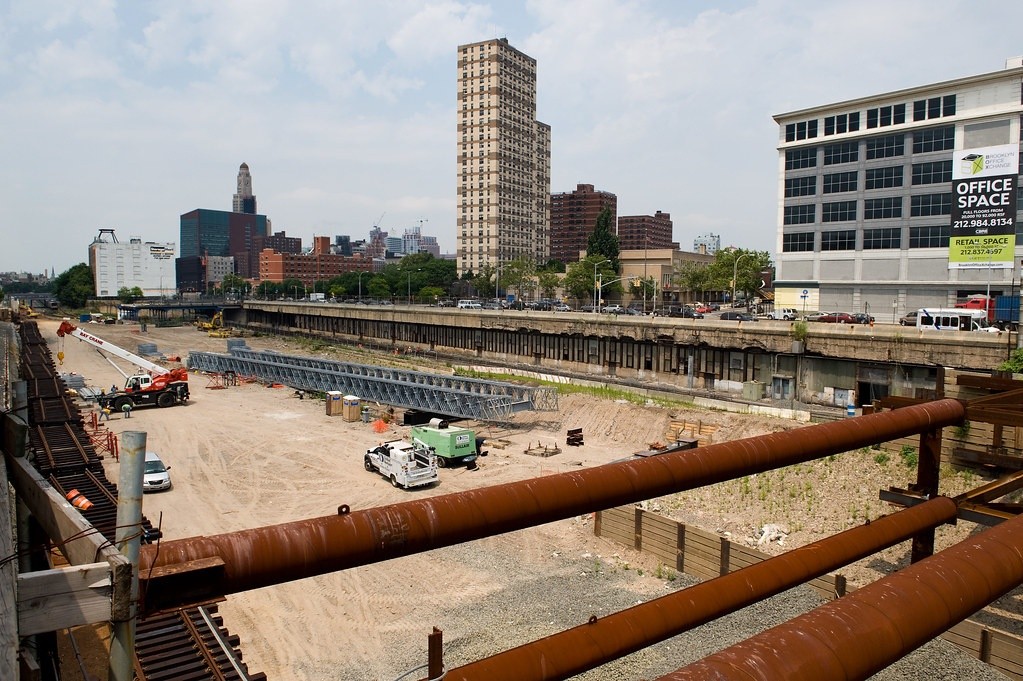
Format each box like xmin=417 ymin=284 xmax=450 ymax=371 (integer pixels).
xmin=768 ymin=307 xmax=797 ymax=321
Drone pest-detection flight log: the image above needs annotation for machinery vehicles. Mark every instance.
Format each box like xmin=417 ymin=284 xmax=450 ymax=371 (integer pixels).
xmin=56 ymin=321 xmax=190 ymax=412
xmin=196 ymin=309 xmax=224 ymax=332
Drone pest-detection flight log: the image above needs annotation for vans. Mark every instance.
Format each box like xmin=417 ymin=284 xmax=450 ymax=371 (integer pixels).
xmin=457 ymin=300 xmax=480 ymax=309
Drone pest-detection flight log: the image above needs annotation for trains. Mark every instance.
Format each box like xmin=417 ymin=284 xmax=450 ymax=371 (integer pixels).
xmin=40 ymin=300 xmax=57 ymax=309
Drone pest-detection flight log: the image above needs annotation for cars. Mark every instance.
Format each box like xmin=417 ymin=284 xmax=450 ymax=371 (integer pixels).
xmin=481 ymin=300 xmax=758 ymax=322
xmin=438 ymin=300 xmax=455 ymax=307
xmin=299 ymin=296 xmax=392 ymax=305
xmin=900 ymin=311 xmax=918 ymax=325
xmin=804 ymin=310 xmax=876 ymax=324
xmin=141 ymin=452 xmax=171 ymax=493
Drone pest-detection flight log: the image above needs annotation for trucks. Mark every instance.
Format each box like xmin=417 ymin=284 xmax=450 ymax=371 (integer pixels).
xmin=307 ymin=293 xmax=325 ymax=301
xmin=917 ymin=307 xmax=1000 ymax=333
xmin=365 ymin=436 xmax=437 ymax=490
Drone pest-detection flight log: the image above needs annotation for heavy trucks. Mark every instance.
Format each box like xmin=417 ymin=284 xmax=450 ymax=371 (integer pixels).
xmin=955 ymin=294 xmax=1020 ymax=332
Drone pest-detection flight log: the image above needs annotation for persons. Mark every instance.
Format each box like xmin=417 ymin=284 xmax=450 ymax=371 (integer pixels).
xmin=749 ymin=304 xmax=762 ymax=313
xmin=122 ymin=395 xmax=133 ymax=418
xmin=358 ymin=342 xmax=363 ymax=349
xmin=394 ymin=346 xmax=420 ymax=356
xmin=300 ymin=389 xmax=306 ymax=399
xmin=98 ymin=399 xmax=111 ymax=421
xmin=736 ymin=314 xmax=743 ymax=321
xmin=100 ymin=387 xmax=106 ymax=396
xmin=111 ymin=384 xmax=118 ymax=392
xmin=132 ymin=378 xmax=140 ymax=390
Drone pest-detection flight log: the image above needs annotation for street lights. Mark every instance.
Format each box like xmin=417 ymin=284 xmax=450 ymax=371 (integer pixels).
xmin=733 ymin=253 xmax=755 ymax=310
xmin=497 ymin=258 xmax=611 ymax=312
xmin=350 ymin=268 xmax=421 ymax=307
xmin=975 ymin=242 xmax=1007 ymax=325
